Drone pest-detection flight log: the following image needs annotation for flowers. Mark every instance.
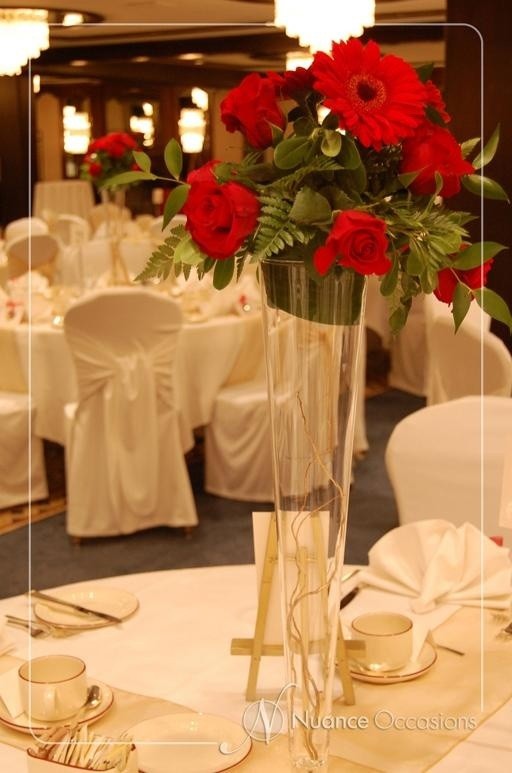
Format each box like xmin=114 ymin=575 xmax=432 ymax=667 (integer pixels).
xmin=77 ymin=39 xmax=512 ymax=330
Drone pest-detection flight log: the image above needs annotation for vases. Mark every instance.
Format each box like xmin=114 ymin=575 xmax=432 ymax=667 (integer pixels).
xmin=259 ymin=260 xmax=365 ymax=768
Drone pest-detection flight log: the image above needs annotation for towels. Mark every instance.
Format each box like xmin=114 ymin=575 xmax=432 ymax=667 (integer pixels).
xmin=361 ymin=517 xmax=511 ymax=615
xmin=0 ymin=629 xmax=19 ymax=658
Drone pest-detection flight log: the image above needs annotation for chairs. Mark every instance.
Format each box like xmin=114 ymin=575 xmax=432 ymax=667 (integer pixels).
xmin=0 ymin=180 xmax=512 ymax=544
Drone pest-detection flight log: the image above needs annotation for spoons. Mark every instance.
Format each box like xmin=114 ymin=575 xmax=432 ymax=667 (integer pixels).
xmin=67 ymin=682 xmax=102 ymax=726
xmin=9 ymin=620 xmax=44 ymax=638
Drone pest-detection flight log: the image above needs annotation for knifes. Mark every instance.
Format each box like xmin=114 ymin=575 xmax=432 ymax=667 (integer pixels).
xmin=29 ymin=590 xmax=122 ymax=624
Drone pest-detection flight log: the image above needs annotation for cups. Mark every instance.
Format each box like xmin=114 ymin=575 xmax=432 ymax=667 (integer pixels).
xmin=351 ymin=612 xmax=414 ymax=671
xmin=18 ymin=655 xmax=88 ymax=722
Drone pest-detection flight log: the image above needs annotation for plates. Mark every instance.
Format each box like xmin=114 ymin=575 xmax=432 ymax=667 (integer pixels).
xmin=334 ymin=639 xmax=438 ymax=686
xmin=0 ymin=680 xmax=114 ymax=736
xmin=33 ymin=584 xmax=139 ymax=627
xmin=122 ymin=714 xmax=252 ymax=771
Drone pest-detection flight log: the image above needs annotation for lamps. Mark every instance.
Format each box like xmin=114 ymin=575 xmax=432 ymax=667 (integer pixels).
xmin=274 ymin=1 xmax=376 ymax=55
xmin=1 ymin=8 xmax=50 ymax=78
